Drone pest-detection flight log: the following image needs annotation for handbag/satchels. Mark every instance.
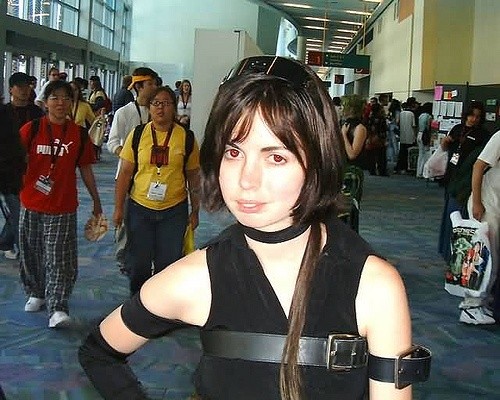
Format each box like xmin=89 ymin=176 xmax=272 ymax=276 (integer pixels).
xmin=407 ymin=145 xmax=419 ymax=171
xmin=423 ymin=147 xmax=449 ymax=178
xmin=87 ymin=118 xmax=106 ymax=146
xmin=444 ymin=211 xmax=492 ymax=298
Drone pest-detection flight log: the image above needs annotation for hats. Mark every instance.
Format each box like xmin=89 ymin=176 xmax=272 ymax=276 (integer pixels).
xmin=10 ymin=72 xmax=33 ymax=86
xmin=127 ymin=75 xmax=156 ymax=91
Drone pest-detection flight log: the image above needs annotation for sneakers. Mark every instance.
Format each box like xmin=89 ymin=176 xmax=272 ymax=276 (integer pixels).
xmin=459 ymin=306 xmax=496 ymax=324
xmin=24 ymin=296 xmax=46 ymax=311
xmin=0 ymin=250 xmax=18 ymax=260
xmin=48 ymin=311 xmax=72 ymax=328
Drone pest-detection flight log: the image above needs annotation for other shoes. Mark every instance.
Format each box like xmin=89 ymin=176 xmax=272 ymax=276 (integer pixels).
xmin=368 ymin=168 xmax=415 ymax=177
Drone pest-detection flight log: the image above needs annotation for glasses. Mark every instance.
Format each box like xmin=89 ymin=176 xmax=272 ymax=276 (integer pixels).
xmin=149 ymin=99 xmax=176 ymax=107
xmin=50 ymin=73 xmax=60 ymax=77
xmin=48 ymin=95 xmax=71 ymax=103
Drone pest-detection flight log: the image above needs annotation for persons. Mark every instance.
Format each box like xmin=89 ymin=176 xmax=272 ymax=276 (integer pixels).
xmin=112 ymin=75 xmax=134 ymax=114
xmin=337 ymin=95 xmax=369 ymax=235
xmin=107 ymin=66 xmax=159 ymax=181
xmin=111 ymin=86 xmax=203 ymax=298
xmin=77 ymin=56 xmax=431 ymax=400
xmin=439 ymin=101 xmax=490 ymax=263
xmin=158 ymin=76 xmax=191 ymax=129
xmin=0 ymin=73 xmax=44 ymax=260
xmin=35 ymin=67 xmax=112 ymax=163
xmin=457 ymin=130 xmax=500 ymax=325
xmin=15 ymin=80 xmax=102 ymax=328
xmin=334 ymin=93 xmax=434 ymax=178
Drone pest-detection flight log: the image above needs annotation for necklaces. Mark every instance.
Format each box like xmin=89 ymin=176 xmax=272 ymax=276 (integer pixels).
xmin=243 ymin=222 xmax=310 ymax=244
